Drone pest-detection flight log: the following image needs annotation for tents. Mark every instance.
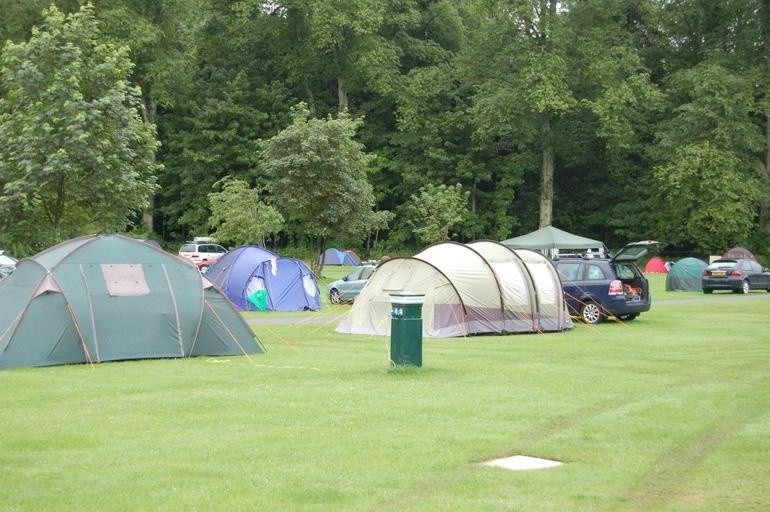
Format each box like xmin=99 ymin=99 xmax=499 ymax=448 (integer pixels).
xmin=0 ymin=233 xmax=265 ymax=370
xmin=666 ymin=256 xmax=710 ymax=291
xmin=318 ymin=247 xmax=361 ymax=266
xmin=644 ymin=256 xmax=666 ymax=273
xmin=0 ymin=248 xmax=18 ymax=283
xmin=722 ymin=247 xmax=754 ymax=260
xmin=203 ymin=244 xmax=321 ymax=311
xmin=333 ymin=238 xmax=574 ymax=338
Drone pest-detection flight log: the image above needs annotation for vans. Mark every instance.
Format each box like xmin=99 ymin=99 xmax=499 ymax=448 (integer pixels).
xmin=551 ymin=239 xmax=669 ymax=326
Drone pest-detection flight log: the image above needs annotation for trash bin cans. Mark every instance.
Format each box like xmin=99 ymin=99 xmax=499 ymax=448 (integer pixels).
xmin=390 ymin=300 xmax=423 ymax=366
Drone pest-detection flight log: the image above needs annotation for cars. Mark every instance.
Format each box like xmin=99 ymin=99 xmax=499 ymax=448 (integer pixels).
xmin=326 ymin=265 xmax=378 ymax=305
xmin=701 ymin=258 xmax=769 ymax=294
xmin=0 ymin=249 xmax=18 ymax=267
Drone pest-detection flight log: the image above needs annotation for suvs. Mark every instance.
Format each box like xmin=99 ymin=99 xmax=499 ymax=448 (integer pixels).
xmin=178 ymin=236 xmax=230 ymax=274
